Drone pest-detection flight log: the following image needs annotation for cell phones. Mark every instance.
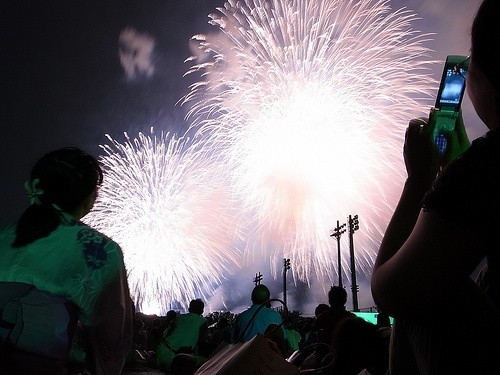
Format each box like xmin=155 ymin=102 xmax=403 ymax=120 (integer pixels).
xmin=427 ymin=55 xmax=469 ymax=156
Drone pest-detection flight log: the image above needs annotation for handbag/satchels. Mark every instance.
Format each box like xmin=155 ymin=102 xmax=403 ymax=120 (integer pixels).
xmin=193 ymin=299 xmax=337 ymax=375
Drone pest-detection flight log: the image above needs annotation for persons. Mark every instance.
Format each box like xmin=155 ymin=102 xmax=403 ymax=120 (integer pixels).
xmin=133 ymin=286 xmax=390 ymax=375
xmin=370 ymin=0 xmax=500 ymax=375
xmin=0 ymin=146 xmax=134 ymax=375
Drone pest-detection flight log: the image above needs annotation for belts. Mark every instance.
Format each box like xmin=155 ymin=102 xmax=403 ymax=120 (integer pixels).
xmin=163 ymin=337 xmax=177 ymax=355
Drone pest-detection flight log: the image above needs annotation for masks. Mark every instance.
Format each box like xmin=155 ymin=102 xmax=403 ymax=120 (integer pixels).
xmin=328 ymin=298 xmax=349 ymax=315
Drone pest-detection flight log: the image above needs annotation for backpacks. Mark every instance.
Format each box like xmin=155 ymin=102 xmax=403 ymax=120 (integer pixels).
xmin=0 ymin=282 xmax=70 ymax=375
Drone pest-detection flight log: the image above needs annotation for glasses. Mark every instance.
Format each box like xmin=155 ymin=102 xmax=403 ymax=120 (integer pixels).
xmin=453 ymin=54 xmax=473 ymax=80
